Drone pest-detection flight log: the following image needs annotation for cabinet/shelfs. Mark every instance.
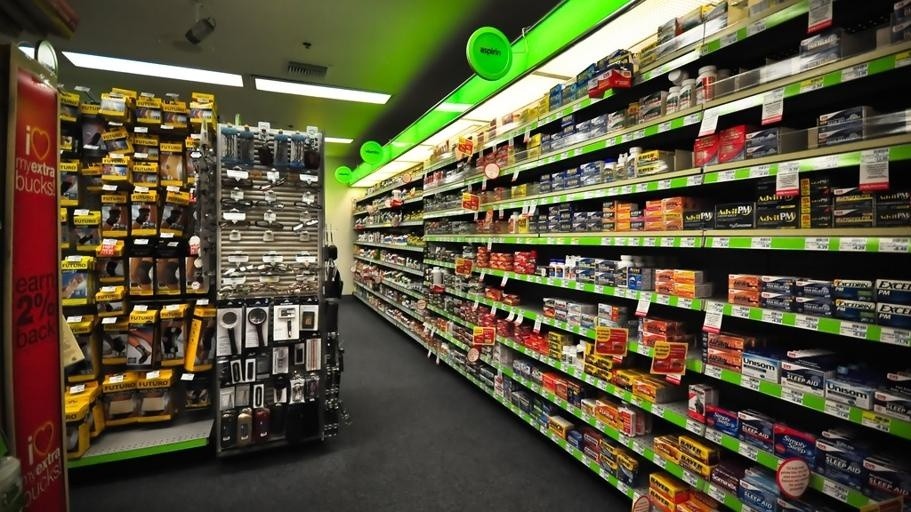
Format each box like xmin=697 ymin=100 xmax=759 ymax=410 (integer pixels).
xmin=351 ymin=2 xmax=911 ymax=512
xmin=59 ymin=84 xmax=344 ymax=472
